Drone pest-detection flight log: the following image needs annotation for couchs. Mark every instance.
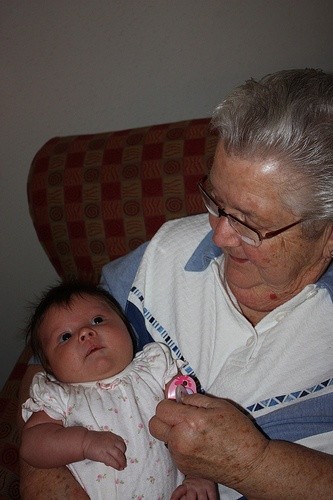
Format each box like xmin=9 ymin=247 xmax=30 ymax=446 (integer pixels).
xmin=0 ymin=117 xmax=224 ymax=500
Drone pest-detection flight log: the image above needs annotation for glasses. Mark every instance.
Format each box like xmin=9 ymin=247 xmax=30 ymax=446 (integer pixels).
xmin=198 ymin=175 xmax=306 ymax=248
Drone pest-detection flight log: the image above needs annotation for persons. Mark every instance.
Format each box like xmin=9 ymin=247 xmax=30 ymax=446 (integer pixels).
xmin=16 ymin=68 xmax=333 ymax=500
xmin=18 ymin=274 xmax=221 ymax=500
